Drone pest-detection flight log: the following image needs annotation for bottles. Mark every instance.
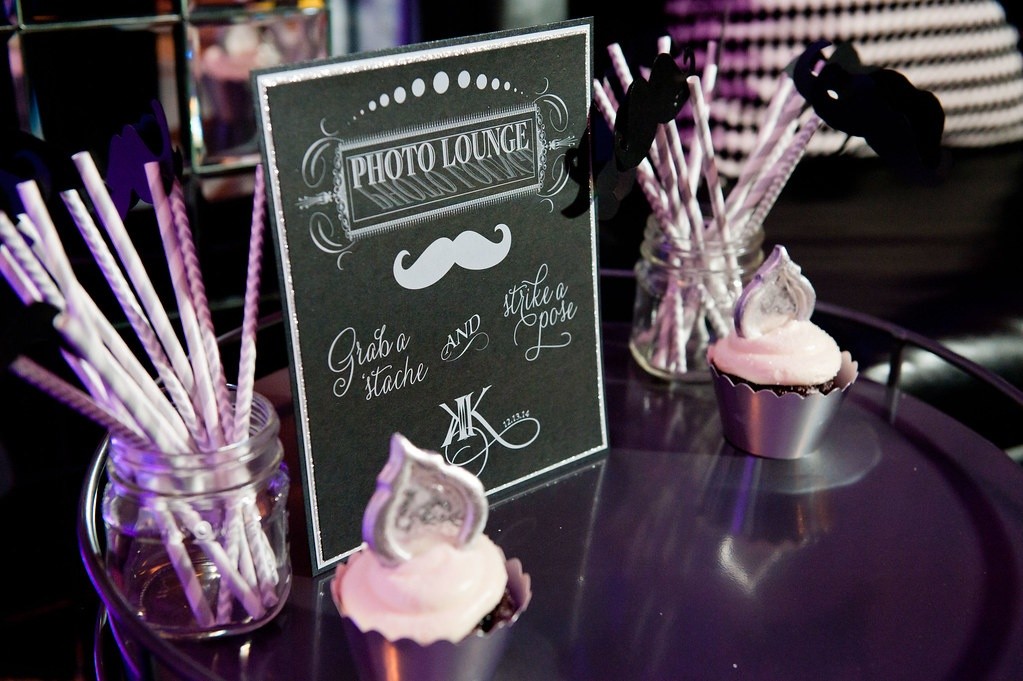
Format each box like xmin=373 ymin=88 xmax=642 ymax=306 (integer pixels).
xmin=628 ymin=211 xmax=764 ymax=388
xmin=101 ymin=381 xmax=290 ymax=640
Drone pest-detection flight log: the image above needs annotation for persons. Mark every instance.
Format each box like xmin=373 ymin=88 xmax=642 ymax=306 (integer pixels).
xmin=662 ymin=1 xmax=1023 ymax=349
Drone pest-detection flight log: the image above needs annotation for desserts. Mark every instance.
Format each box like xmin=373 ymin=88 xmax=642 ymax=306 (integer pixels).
xmin=705 ymin=243 xmax=859 ymax=461
xmin=327 ymin=433 xmax=531 ymax=681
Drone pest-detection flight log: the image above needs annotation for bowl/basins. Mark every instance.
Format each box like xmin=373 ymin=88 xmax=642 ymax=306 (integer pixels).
xmin=330 ymin=534 xmax=532 ymax=681
xmin=705 ymin=341 xmax=859 ymax=460
